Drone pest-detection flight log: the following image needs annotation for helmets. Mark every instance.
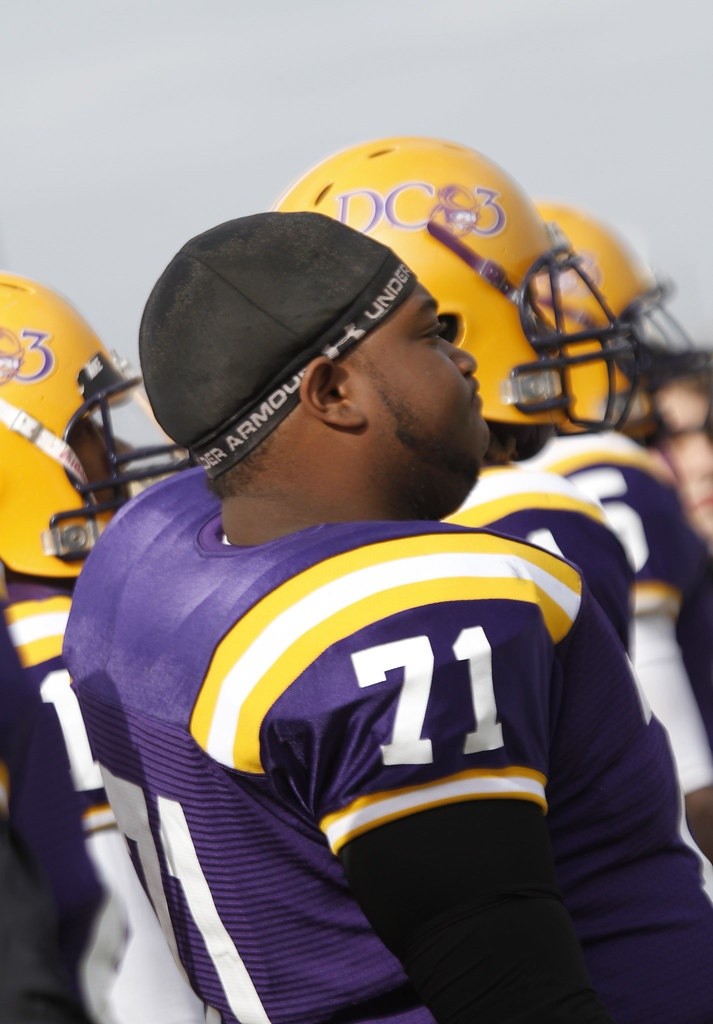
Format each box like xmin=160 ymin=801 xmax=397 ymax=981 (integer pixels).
xmin=275 ymin=137 xmax=639 ymax=437
xmin=0 ymin=273 xmax=193 ymax=576
xmin=530 ymin=203 xmax=708 ymax=423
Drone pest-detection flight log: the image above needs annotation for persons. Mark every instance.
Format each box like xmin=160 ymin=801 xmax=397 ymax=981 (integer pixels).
xmin=0 ymin=133 xmax=713 ymax=1024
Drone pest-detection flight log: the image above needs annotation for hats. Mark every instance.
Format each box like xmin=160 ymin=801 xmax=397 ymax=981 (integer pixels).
xmin=139 ymin=213 xmax=418 ymax=473
